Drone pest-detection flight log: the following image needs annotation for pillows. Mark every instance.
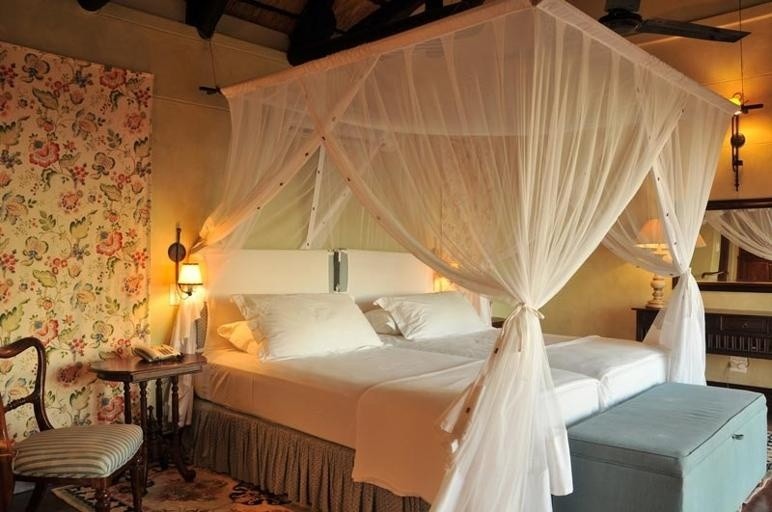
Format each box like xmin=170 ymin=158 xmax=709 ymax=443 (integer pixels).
xmin=373 ymin=290 xmax=489 ymax=339
xmin=364 ymin=309 xmax=401 ymax=335
xmin=216 ymin=321 xmax=259 ymax=355
xmin=229 ymin=292 xmax=382 ymax=364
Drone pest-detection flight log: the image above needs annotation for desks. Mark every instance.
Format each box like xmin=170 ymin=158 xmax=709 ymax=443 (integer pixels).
xmin=631 ymin=308 xmax=772 ymax=361
xmin=88 ymin=354 xmax=206 ymax=497
xmin=491 ymin=316 xmax=506 ymax=330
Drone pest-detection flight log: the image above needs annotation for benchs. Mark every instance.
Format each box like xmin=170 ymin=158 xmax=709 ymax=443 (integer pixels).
xmin=552 ymin=381 xmax=768 ymax=512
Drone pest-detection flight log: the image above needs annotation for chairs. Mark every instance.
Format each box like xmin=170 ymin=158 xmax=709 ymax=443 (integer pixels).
xmin=0 ymin=338 xmax=145 ymax=512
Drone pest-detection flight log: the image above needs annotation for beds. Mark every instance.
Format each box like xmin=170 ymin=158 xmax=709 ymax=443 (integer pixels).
xmin=181 ymin=246 xmax=667 ymax=512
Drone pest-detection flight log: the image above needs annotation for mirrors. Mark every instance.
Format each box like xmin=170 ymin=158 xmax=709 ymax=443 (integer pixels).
xmin=671 ymin=198 xmax=772 ymax=293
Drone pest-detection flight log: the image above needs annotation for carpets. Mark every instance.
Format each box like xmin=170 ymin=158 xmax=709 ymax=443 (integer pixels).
xmin=48 ymin=464 xmax=310 ymax=512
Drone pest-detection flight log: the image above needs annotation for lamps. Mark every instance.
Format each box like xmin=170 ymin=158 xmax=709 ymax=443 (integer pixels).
xmin=724 ymin=1 xmax=763 ymax=192
xmin=168 ymin=228 xmax=203 ymax=296
xmin=634 ymin=219 xmax=680 ymax=309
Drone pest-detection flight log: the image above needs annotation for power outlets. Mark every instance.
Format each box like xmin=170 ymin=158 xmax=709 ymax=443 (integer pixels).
xmin=729 ymin=356 xmax=748 ymax=373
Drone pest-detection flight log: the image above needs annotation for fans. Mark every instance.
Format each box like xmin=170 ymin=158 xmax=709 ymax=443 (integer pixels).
xmin=527 ymin=0 xmax=751 ymax=49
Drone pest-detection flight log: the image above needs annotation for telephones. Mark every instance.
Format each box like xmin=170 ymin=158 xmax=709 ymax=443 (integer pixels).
xmin=133 ymin=343 xmax=181 ymax=363
